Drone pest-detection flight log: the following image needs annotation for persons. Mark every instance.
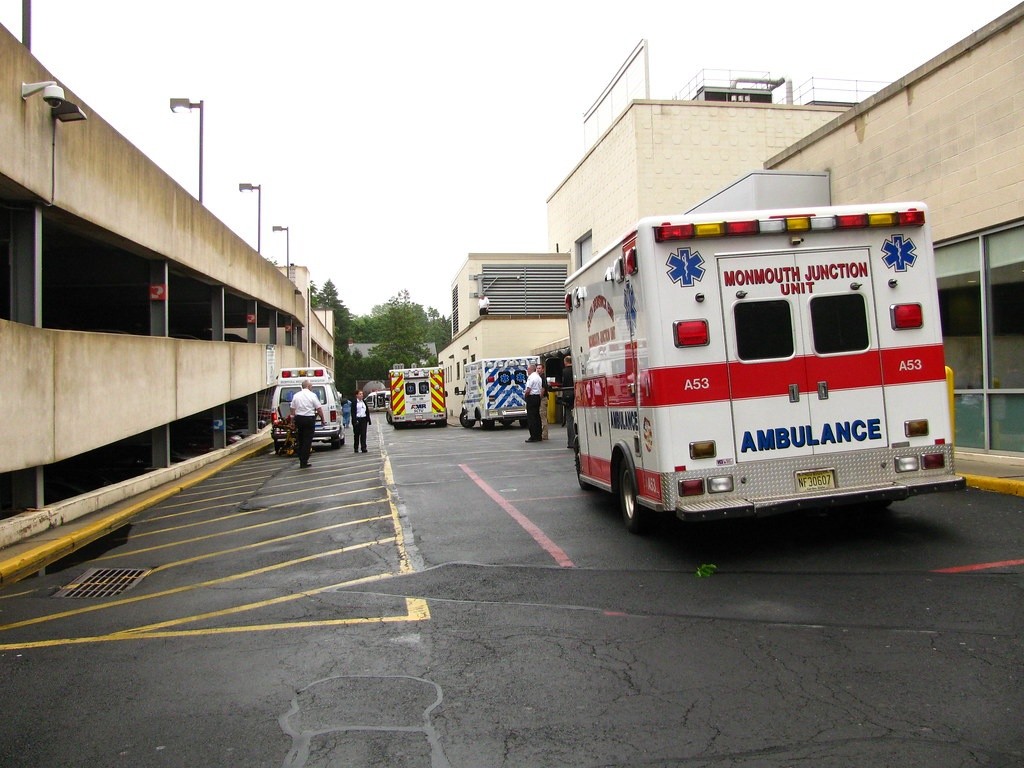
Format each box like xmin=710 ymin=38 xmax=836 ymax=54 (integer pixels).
xmin=562 ymin=356 xmax=577 ymax=448
xmin=477 ymin=294 xmax=489 ymax=315
xmin=585 ymin=380 xmax=607 ymax=407
xmin=523 ymin=364 xmax=542 ymax=442
xmin=351 ymin=390 xmax=371 ymax=453
xmin=290 ymin=380 xmax=325 ymax=467
xmin=342 ymin=399 xmax=352 ymax=426
xmin=537 ymin=365 xmax=549 ymax=440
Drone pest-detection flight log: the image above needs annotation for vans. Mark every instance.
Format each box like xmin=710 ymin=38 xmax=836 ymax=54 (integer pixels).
xmin=364 ymin=391 xmax=391 ymax=411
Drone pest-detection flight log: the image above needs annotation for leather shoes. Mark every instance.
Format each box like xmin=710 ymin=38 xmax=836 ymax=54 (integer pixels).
xmin=525 ymin=435 xmax=543 ymax=443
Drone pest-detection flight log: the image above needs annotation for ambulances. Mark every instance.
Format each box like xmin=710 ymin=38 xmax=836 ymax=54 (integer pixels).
xmin=454 ymin=355 xmax=540 ymax=430
xmin=386 ymin=367 xmax=449 ymax=431
xmin=540 ymin=199 xmax=968 ymax=537
xmin=270 ymin=366 xmax=346 ymax=454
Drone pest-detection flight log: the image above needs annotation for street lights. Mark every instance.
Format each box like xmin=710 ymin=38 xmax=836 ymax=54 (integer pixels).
xmin=170 ymin=98 xmax=204 ymax=202
xmin=273 ymin=226 xmax=289 ymax=278
xmin=238 ymin=183 xmax=261 ymax=253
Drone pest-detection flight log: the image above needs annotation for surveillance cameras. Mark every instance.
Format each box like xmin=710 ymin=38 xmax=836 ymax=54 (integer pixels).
xmin=42 ymin=86 xmax=66 ymax=108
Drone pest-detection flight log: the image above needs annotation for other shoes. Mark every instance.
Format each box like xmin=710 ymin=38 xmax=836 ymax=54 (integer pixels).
xmin=299 ymin=458 xmax=312 ymax=469
xmin=353 ymin=446 xmax=367 ymax=453
